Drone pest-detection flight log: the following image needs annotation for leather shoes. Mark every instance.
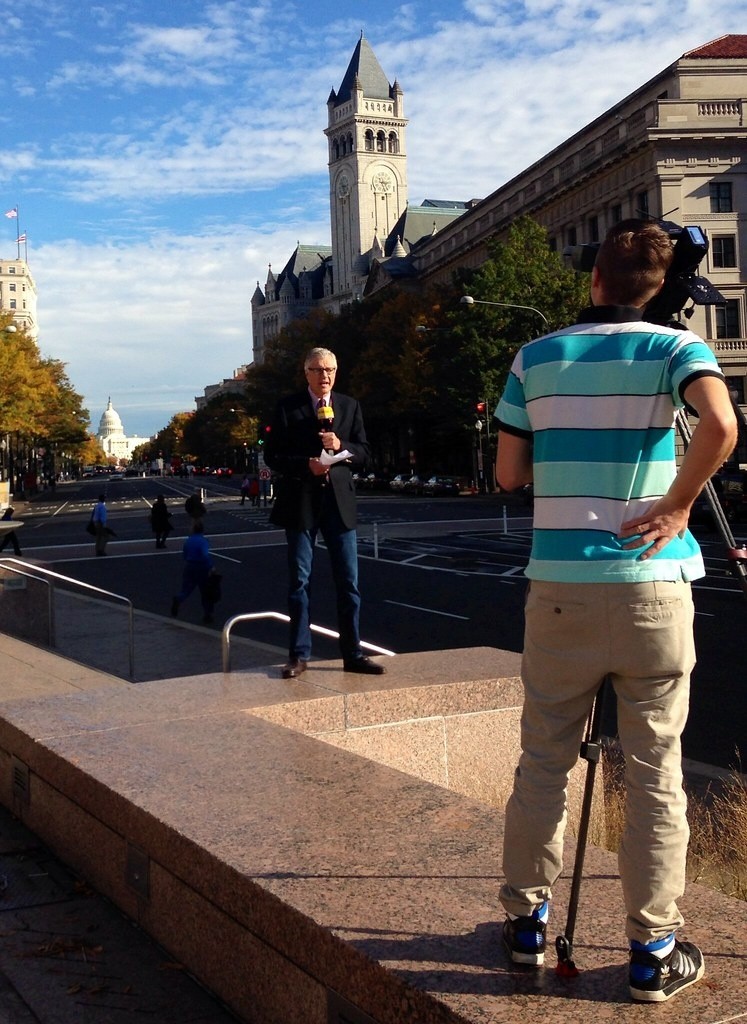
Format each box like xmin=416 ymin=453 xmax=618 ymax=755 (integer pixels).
xmin=281 ymin=658 xmax=307 ymax=678
xmin=344 ymin=654 xmax=387 ymax=675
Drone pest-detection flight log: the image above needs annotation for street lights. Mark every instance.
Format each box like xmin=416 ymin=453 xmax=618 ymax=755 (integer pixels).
xmin=475 ymin=419 xmax=486 ymax=495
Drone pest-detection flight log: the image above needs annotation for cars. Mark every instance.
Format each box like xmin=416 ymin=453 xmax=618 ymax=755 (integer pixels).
xmin=109 ymin=470 xmax=125 ymax=481
xmin=82 ymin=462 xmax=206 ymax=480
xmin=389 ymin=473 xmax=461 ymax=498
xmin=210 ymin=467 xmax=232 ymax=481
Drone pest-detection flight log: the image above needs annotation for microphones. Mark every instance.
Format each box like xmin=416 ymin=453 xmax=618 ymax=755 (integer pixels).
xmin=317 ymin=399 xmax=335 ymax=457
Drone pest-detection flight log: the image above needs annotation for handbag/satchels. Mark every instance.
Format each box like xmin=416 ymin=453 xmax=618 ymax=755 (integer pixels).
xmin=146 ymin=510 xmax=152 ymax=520
xmin=185 ymin=499 xmax=191 ymax=515
xmin=84 ymin=519 xmax=97 ymax=536
xmin=201 ymin=573 xmax=222 ymax=604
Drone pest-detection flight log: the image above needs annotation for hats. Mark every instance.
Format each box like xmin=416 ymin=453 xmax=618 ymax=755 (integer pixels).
xmin=194 ymin=487 xmax=201 ymax=492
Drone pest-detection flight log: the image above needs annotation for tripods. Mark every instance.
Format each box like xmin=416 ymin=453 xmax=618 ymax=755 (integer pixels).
xmin=555 ymin=405 xmax=747 ymax=973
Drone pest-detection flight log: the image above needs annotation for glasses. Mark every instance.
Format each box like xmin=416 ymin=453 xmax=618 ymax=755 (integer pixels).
xmin=308 ymin=367 xmax=335 ymax=375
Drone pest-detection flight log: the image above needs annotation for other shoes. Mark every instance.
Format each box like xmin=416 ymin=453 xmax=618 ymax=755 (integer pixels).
xmin=204 ymin=615 xmax=215 ymax=624
xmin=96 ymin=552 xmax=108 ymax=556
xmin=171 ymin=596 xmax=180 ymax=618
xmin=156 ymin=543 xmax=167 ymax=549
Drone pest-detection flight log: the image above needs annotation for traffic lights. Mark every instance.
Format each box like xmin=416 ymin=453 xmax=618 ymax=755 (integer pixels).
xmin=264 ymin=425 xmax=273 ymax=433
xmin=257 ymin=438 xmax=265 ymax=446
xmin=477 ymin=403 xmax=485 ymax=412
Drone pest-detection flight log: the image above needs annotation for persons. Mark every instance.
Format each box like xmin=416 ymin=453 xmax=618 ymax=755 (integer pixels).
xmin=495 ymin=217 xmax=738 ymax=1001
xmin=0 ymin=508 xmax=22 ymax=556
xmin=191 ymin=490 xmax=205 ymax=526
xmin=171 ymin=521 xmax=215 ymax=623
xmin=151 ymin=495 xmax=175 ymax=549
xmin=94 ymin=495 xmax=107 ymax=556
xmin=264 ymin=349 xmax=386 ymax=677
xmin=240 ymin=476 xmax=259 ymax=506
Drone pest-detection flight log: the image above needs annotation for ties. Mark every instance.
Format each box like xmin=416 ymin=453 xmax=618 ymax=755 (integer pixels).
xmin=317 ymin=398 xmax=327 ymax=407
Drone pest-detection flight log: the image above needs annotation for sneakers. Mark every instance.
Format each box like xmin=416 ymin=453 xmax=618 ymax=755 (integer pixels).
xmin=629 ymin=934 xmax=705 ymax=1001
xmin=503 ymin=901 xmax=548 ymax=965
